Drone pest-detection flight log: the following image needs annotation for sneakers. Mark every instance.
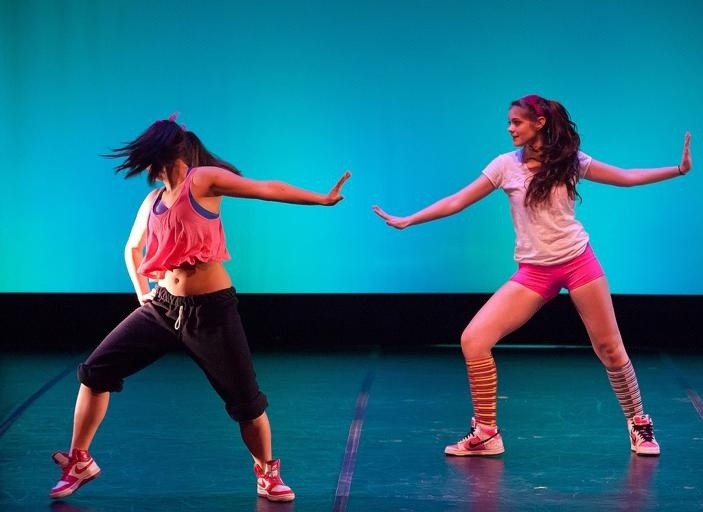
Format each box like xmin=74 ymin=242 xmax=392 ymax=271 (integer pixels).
xmin=253 ymin=457 xmax=296 ymax=503
xmin=442 ymin=415 xmax=507 ymax=457
xmin=49 ymin=447 xmax=102 ymax=500
xmin=627 ymin=413 xmax=662 ymax=457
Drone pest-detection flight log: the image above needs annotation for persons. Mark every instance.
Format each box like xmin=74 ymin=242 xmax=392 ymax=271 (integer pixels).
xmin=49 ymin=119 xmax=352 ymax=502
xmin=372 ymin=96 xmax=691 ymax=459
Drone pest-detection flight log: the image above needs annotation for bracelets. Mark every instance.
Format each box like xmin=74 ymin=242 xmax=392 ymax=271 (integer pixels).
xmin=678 ymin=165 xmax=683 ymax=175
